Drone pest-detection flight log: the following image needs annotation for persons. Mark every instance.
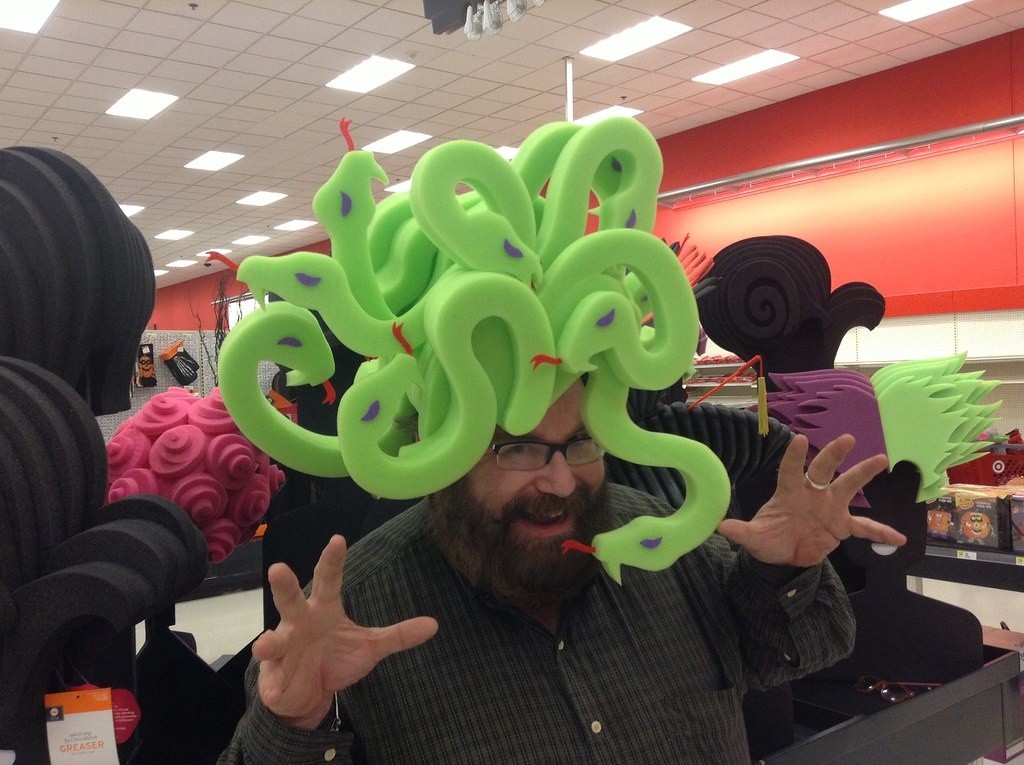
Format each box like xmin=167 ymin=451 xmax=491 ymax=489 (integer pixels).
xmin=215 ymin=371 xmax=906 ymax=765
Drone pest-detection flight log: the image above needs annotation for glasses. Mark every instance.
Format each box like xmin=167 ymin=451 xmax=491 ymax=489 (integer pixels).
xmin=488 ymin=436 xmax=601 ymax=470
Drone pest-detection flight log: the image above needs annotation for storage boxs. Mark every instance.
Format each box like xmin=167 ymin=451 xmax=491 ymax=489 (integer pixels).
xmin=923 ymin=453 xmax=1024 ymax=551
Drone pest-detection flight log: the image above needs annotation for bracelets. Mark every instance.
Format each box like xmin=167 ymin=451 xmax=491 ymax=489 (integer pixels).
xmin=328 ymin=691 xmax=343 ymax=732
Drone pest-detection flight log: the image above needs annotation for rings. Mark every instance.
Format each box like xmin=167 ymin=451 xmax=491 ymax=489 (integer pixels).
xmin=805 ymin=472 xmax=830 ymax=490
